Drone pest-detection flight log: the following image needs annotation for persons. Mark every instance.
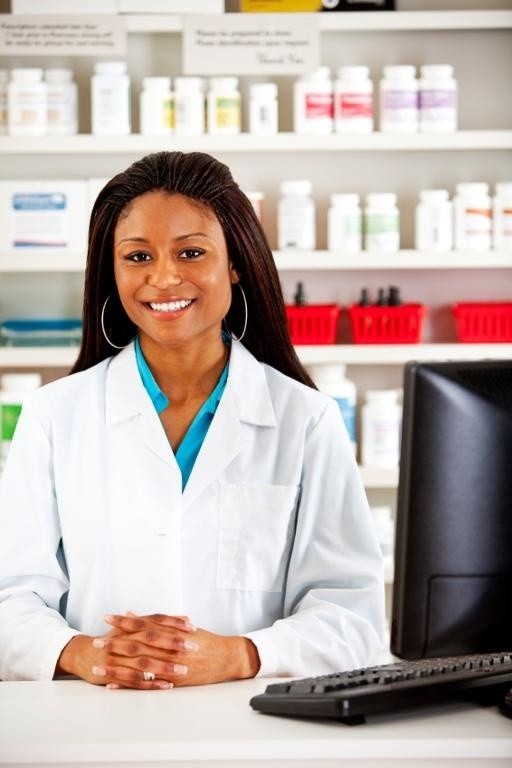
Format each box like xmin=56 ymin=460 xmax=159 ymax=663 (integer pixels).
xmin=0 ymin=150 xmax=387 ymax=693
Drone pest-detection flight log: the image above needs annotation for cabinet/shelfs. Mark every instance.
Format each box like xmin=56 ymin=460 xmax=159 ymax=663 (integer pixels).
xmin=3 ymin=4 xmax=512 ymax=585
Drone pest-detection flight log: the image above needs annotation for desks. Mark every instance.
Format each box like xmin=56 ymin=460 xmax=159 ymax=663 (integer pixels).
xmin=1 ymin=667 xmax=512 ymax=766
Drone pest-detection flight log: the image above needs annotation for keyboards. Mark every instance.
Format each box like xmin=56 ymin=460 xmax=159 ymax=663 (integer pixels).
xmin=250 ymin=652 xmax=512 ymax=726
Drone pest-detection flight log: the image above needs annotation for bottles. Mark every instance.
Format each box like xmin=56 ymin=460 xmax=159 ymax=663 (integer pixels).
xmin=276 ymin=176 xmax=512 ymax=261
xmin=293 ymin=282 xmax=306 ymax=304
xmin=359 ymin=285 xmax=401 ymax=306
xmin=0 ymin=373 xmax=44 ymax=462
xmin=7 ymin=63 xmax=462 ymax=139
xmin=310 ymin=367 xmax=402 ymax=467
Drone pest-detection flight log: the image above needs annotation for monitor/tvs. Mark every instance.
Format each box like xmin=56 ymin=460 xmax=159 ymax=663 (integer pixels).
xmin=389 ymin=360 xmax=512 ymax=720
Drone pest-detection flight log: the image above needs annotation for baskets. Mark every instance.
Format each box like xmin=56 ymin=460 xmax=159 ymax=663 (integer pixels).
xmin=281 ymin=305 xmax=339 ymax=344
xmin=451 ymin=301 xmax=512 ymax=343
xmin=349 ymin=303 xmax=427 ymax=344
